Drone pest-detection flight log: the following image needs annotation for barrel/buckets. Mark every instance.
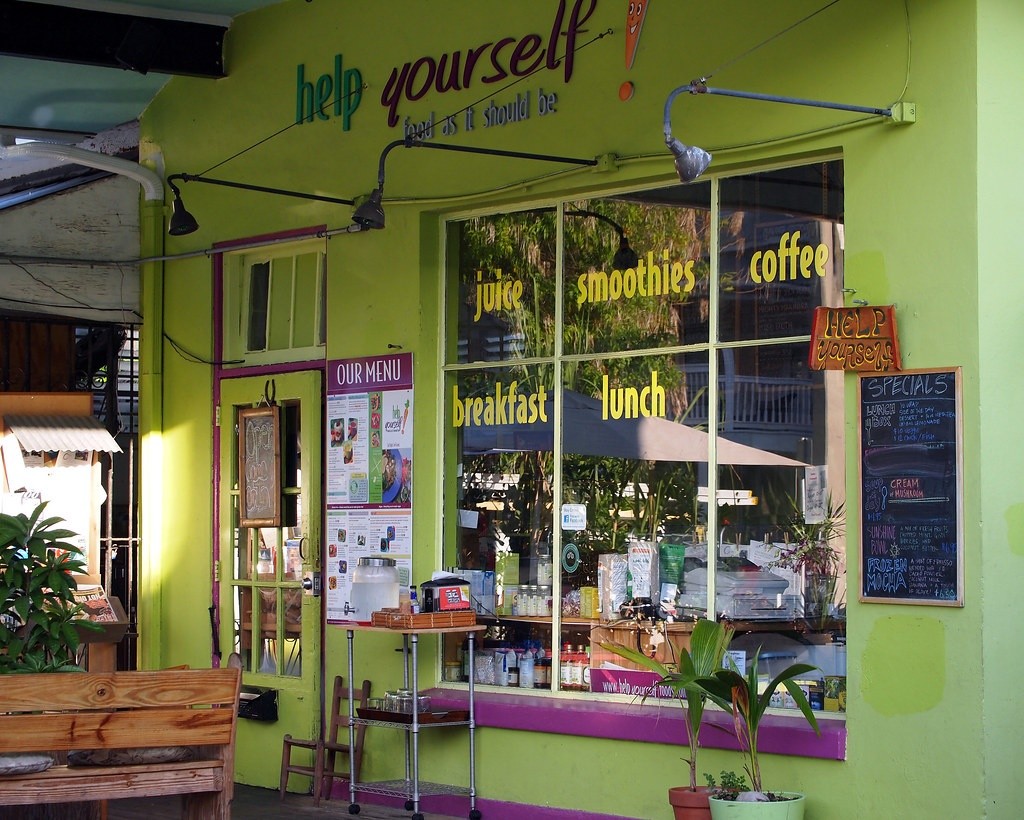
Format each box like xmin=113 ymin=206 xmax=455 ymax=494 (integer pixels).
xmin=343 ymin=557 xmax=400 ymax=626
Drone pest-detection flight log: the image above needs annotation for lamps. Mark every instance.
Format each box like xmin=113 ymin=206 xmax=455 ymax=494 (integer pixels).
xmin=352 ymin=189 xmax=387 ymax=230
xmin=169 ymin=199 xmax=200 ymax=238
xmin=666 ymin=135 xmax=714 ymax=185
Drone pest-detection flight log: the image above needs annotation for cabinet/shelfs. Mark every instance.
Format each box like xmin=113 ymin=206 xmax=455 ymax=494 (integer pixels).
xmin=327 ymin=614 xmax=489 ymax=820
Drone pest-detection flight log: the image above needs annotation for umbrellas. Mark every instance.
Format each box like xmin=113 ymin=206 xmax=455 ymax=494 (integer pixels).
xmin=462 ymin=386 xmax=811 ymax=468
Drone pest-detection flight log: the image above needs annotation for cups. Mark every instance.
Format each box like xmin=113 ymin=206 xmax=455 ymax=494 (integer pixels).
xmin=367 ymin=688 xmax=431 ymax=713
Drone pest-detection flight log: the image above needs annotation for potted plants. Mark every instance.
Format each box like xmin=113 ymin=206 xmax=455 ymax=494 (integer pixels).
xmin=638 ymin=638 xmax=826 ymax=819
xmin=585 ymin=614 xmax=741 ymax=820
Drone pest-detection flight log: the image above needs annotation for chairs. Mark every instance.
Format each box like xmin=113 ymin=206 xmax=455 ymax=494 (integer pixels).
xmin=278 ymin=675 xmax=372 ymax=807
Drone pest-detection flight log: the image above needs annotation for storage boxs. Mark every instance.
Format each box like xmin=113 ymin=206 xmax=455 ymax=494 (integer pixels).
xmin=755 ymin=650 xmax=801 ymax=681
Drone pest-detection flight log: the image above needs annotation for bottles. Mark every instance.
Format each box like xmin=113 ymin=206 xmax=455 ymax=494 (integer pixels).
xmin=519 ymin=640 xmax=544 ymax=687
xmin=461 ymin=632 xmax=479 ymax=683
xmin=408 ymin=585 xmax=420 ymax=614
xmin=561 ymin=644 xmax=590 ymax=691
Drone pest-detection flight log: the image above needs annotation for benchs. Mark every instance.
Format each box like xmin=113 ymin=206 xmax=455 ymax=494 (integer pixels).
xmin=1 ymin=653 xmax=243 ymax=820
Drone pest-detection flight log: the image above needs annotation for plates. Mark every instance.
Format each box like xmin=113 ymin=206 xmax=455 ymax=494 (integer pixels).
xmin=383 ymin=449 xmax=402 ymax=503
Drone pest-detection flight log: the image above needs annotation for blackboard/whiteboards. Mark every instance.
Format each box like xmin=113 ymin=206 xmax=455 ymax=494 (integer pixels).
xmin=239 ymin=406 xmax=279 ymax=526
xmin=855 ymin=366 xmax=966 ymax=607
